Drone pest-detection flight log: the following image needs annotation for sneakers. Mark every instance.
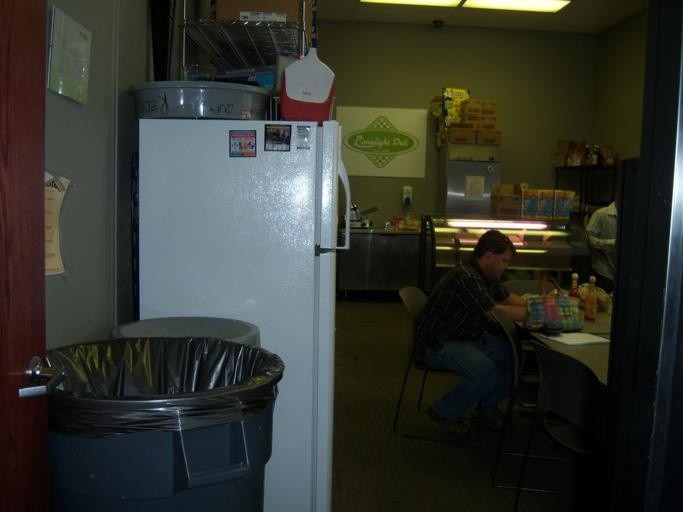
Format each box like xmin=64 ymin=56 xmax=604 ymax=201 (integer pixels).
xmin=425 ymin=407 xmax=473 ymax=436
xmin=473 ymin=409 xmax=515 ymax=429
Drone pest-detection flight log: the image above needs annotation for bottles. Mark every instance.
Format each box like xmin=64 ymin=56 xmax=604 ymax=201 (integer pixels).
xmin=567 ymin=139 xmax=614 ymax=167
xmin=584 ymin=275 xmax=597 ymax=321
xmin=568 ymin=273 xmax=581 ymax=296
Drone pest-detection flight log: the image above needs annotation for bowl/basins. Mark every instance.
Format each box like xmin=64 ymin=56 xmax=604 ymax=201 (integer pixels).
xmin=125 ymin=80 xmax=274 ymax=119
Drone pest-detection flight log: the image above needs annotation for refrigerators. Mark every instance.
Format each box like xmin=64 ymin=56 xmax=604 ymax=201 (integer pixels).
xmin=131 ymin=119 xmax=350 ymax=512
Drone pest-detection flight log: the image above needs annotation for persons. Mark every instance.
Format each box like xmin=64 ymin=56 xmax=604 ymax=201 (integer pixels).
xmin=406 ymin=228 xmax=560 ymax=441
xmin=582 ymin=200 xmax=618 ymax=298
xmin=265 ymin=126 xmax=290 ymax=146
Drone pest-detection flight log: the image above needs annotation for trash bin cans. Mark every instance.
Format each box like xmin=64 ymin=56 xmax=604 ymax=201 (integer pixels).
xmin=114 ymin=317 xmax=261 ymax=348
xmin=44 ymin=336 xmax=285 ymax=512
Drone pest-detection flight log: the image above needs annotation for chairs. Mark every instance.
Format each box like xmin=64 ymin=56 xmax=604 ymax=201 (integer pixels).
xmin=514 ymin=339 xmax=612 ymax=509
xmin=501 ymin=278 xmax=563 ymax=409
xmin=491 ymin=306 xmax=586 ymax=501
xmin=395 ymin=285 xmax=490 ymax=441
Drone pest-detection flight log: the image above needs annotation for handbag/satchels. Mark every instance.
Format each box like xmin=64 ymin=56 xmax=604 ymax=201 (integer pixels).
xmin=520 ymin=276 xmax=584 ymax=335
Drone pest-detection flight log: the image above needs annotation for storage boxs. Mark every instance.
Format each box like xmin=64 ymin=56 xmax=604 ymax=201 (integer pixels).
xmin=442 ymin=95 xmax=502 ymax=146
xmin=488 ymin=180 xmax=528 ymax=220
